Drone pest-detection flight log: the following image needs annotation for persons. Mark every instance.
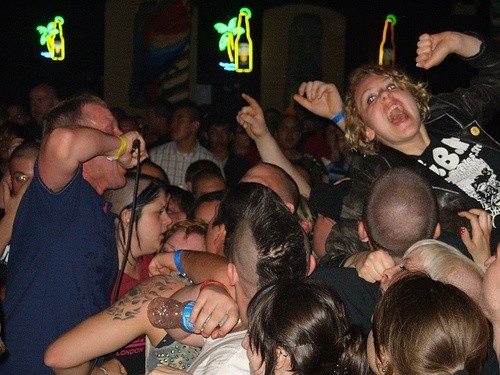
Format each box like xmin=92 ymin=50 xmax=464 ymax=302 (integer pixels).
xmin=0 ymin=31 xmax=500 ymax=375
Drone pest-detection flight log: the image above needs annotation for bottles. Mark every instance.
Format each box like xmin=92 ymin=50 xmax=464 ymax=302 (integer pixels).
xmin=147 ymin=296 xmax=228 ymax=333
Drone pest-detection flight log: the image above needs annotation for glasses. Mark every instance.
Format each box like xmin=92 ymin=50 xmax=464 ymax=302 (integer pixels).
xmin=13 ymin=174 xmax=31 ymax=185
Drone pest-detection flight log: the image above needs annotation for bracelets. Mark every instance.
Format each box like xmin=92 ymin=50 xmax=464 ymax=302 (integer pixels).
xmin=198 ymin=279 xmax=229 ymax=292
xmin=173 ymin=250 xmax=185 ymax=274
xmin=331 ymin=112 xmax=346 ymax=124
xmin=106 ymin=136 xmax=127 ymax=160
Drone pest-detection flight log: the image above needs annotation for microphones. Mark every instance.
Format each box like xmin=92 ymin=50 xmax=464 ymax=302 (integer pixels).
xmin=131 ymin=139 xmax=140 ymax=153
